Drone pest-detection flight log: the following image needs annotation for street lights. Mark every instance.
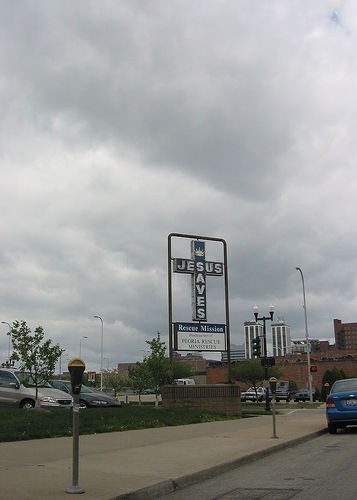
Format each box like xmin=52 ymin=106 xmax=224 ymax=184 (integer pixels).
xmin=80 ymin=336 xmax=89 ymax=359
xmin=2 ymin=322 xmax=11 ymax=357
xmin=252 ymin=302 xmax=275 ymax=388
xmin=93 ymin=315 xmax=104 ymax=392
xmin=59 ymin=355 xmax=69 ymax=374
xmin=296 ymin=267 xmax=313 ymax=402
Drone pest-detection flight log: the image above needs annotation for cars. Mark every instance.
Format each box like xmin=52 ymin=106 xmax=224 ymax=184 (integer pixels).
xmin=143 ymin=389 xmax=160 ymax=394
xmin=0 ymin=368 xmax=74 ymax=410
xmin=240 ymin=386 xmax=268 ymax=402
xmin=294 ymin=386 xmax=331 ymax=402
xmin=45 ymin=380 xmax=122 ymax=408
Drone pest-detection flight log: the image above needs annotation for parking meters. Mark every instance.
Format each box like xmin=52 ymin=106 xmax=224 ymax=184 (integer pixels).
xmin=269 ymin=377 xmax=279 ymax=439
xmin=65 ymin=357 xmax=85 ymax=494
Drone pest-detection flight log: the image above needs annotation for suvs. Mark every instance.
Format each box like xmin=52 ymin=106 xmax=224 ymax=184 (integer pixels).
xmin=267 ymin=379 xmax=298 ymax=402
xmin=325 ymin=378 xmax=357 ymax=434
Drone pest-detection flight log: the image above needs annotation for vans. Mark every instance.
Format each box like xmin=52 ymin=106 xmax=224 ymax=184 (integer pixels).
xmin=172 ymin=378 xmax=196 ymax=384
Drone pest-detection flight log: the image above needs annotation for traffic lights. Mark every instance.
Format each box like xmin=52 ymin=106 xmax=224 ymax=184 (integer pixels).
xmin=310 ymin=365 xmax=318 ymax=372
xmin=252 ymin=337 xmax=261 ymax=356
xmin=261 ymin=357 xmax=272 ymax=367
